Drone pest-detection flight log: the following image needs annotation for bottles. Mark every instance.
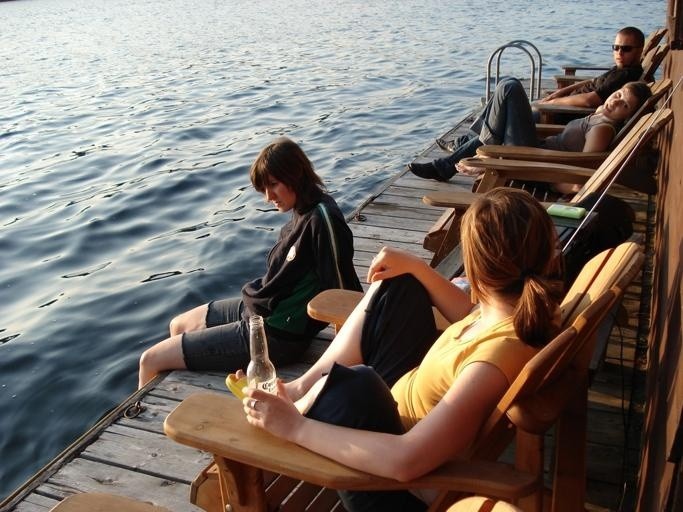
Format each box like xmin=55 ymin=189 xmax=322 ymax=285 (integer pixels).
xmin=245 ymin=315 xmax=277 ymax=400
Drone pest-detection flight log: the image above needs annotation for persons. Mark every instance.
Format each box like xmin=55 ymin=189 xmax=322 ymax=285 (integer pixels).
xmin=455 ymin=77 xmax=656 ymax=195
xmin=408 ymin=26 xmax=646 ymax=182
xmin=224 ymin=186 xmax=568 ymax=512
xmin=136 ymin=137 xmax=365 ymax=391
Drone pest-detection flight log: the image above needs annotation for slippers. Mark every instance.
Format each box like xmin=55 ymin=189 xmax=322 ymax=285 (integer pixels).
xmin=226 ymin=374 xmax=247 ymax=401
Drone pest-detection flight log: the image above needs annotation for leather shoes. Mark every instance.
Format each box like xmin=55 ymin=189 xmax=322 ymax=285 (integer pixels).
xmin=408 ymin=161 xmax=445 ymax=182
xmin=436 ymin=139 xmax=453 ymax=153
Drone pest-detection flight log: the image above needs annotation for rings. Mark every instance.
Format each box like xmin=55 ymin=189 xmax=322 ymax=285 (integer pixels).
xmin=250 ymin=399 xmax=257 ymax=410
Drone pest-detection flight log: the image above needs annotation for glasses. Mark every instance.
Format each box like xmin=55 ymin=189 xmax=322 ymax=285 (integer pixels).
xmin=612 ymin=45 xmax=641 ymax=52
